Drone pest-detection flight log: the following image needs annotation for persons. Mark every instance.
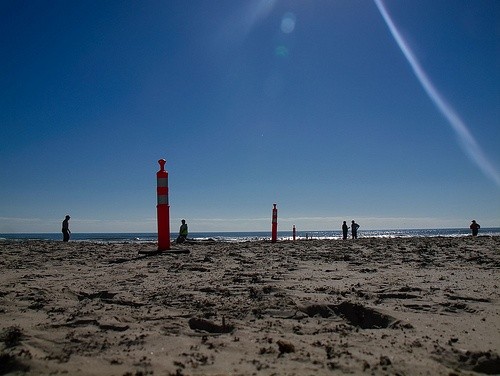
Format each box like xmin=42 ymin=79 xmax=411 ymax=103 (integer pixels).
xmin=350 ymin=220 xmax=361 ymax=240
xmin=178 ymin=219 xmax=190 ymax=245
xmin=342 ymin=221 xmax=351 ymax=241
xmin=471 ymin=220 xmax=480 ymax=236
xmin=62 ymin=215 xmax=73 ymax=243
